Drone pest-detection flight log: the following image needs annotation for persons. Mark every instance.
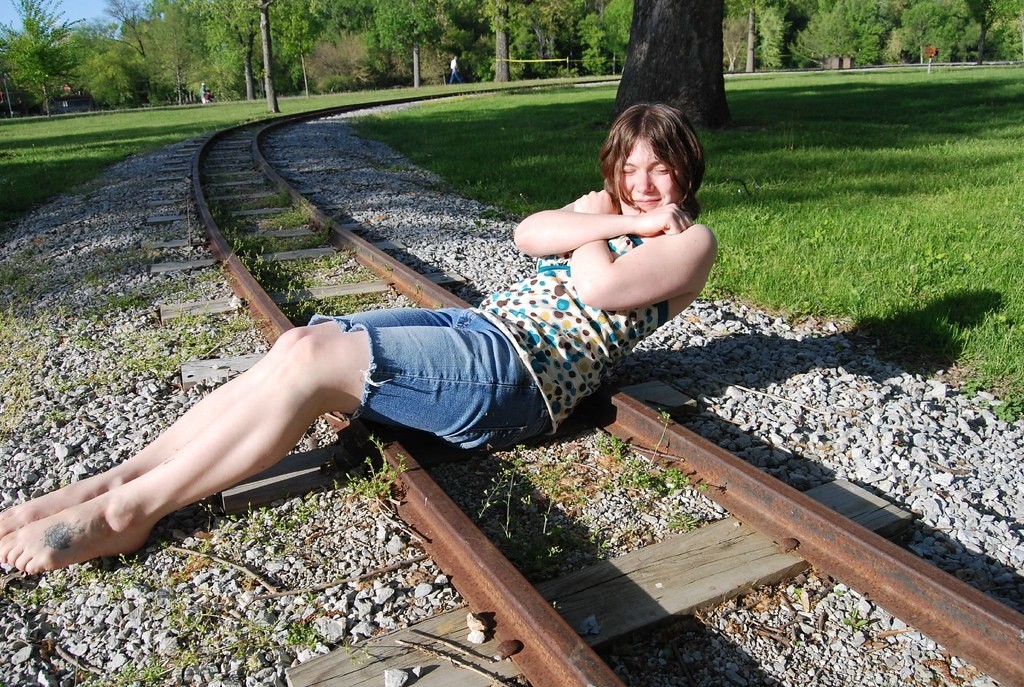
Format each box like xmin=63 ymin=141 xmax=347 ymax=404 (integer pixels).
xmin=450 ymin=56 xmax=462 ymax=83
xmin=0 ymin=102 xmax=717 ymax=573
xmin=200 ymin=83 xmax=214 ymax=105
